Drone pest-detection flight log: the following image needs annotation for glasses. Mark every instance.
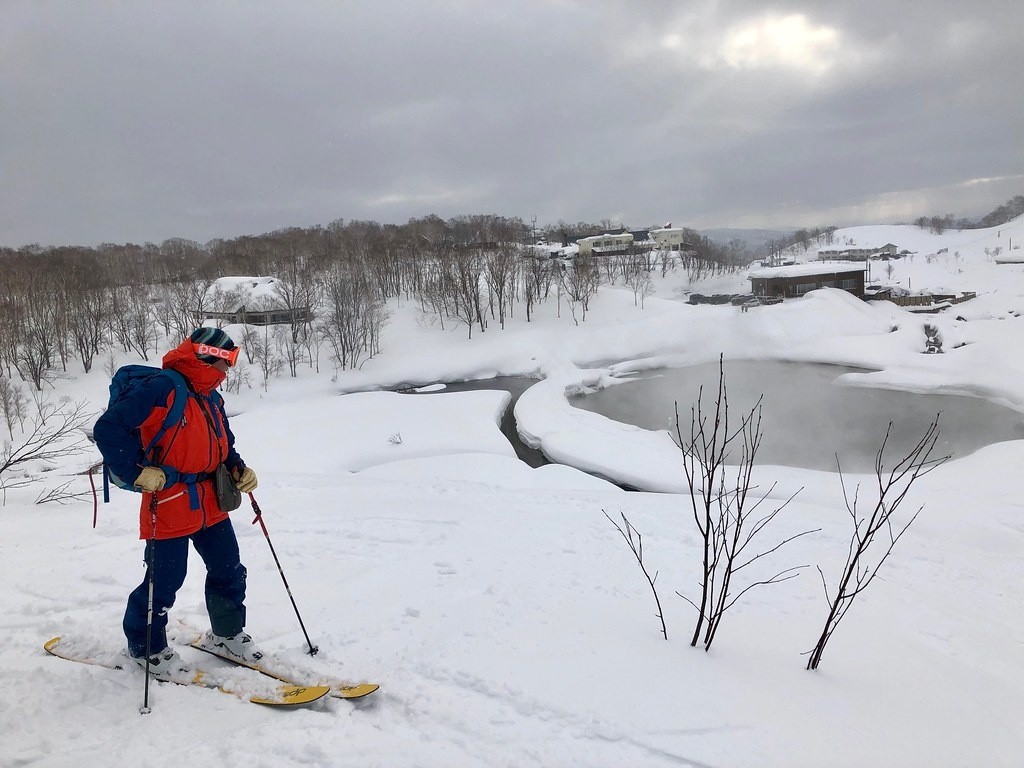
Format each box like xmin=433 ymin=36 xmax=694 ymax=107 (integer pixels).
xmin=226 ymin=346 xmax=240 ymax=367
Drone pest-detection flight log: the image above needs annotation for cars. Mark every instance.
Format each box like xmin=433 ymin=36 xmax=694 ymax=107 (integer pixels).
xmin=742 ymin=299 xmax=760 ymax=307
xmin=755 ymin=296 xmax=783 ymax=305
xmin=732 ymin=295 xmax=754 ymax=306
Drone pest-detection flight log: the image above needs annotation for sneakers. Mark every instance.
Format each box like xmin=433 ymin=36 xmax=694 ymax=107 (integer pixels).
xmin=129 ymin=647 xmax=190 ymax=685
xmin=209 ymin=632 xmax=256 ymax=657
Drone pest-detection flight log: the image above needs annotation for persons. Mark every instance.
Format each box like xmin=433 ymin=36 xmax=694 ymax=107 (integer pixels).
xmin=94 ymin=328 xmax=262 ymax=673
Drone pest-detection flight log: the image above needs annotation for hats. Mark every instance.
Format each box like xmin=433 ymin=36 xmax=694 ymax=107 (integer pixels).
xmin=191 ymin=327 xmax=234 ymax=365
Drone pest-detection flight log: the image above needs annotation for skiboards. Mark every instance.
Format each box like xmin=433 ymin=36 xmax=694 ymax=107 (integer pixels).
xmin=43 ymin=618 xmax=382 ymax=709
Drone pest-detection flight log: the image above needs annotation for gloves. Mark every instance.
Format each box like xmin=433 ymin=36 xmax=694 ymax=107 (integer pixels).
xmin=134 ymin=466 xmax=166 ymax=493
xmin=232 ymin=466 xmax=258 ymax=493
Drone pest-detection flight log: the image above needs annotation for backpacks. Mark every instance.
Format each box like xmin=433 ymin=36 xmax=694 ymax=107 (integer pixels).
xmin=103 ymin=365 xmax=223 ymax=510
